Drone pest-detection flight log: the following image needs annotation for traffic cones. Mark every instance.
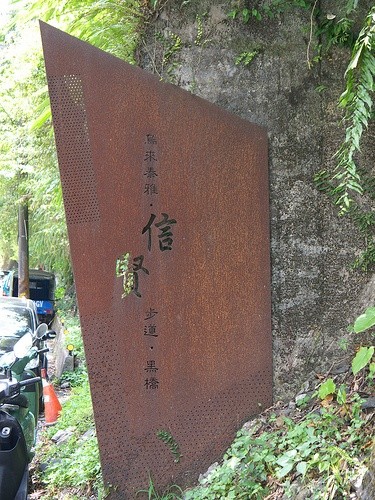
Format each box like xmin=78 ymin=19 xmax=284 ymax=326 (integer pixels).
xmin=38 ymin=368 xmax=62 ymax=429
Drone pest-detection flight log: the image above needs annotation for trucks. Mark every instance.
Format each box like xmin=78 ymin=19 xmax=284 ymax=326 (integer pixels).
xmin=0 ymin=267 xmax=57 ymax=329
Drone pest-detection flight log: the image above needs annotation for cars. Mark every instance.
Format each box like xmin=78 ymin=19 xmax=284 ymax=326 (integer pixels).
xmin=0 ymin=296 xmax=56 ymax=413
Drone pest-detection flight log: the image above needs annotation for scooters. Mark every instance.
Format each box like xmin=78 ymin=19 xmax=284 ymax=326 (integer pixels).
xmin=0 ymin=323 xmax=50 ymax=500
